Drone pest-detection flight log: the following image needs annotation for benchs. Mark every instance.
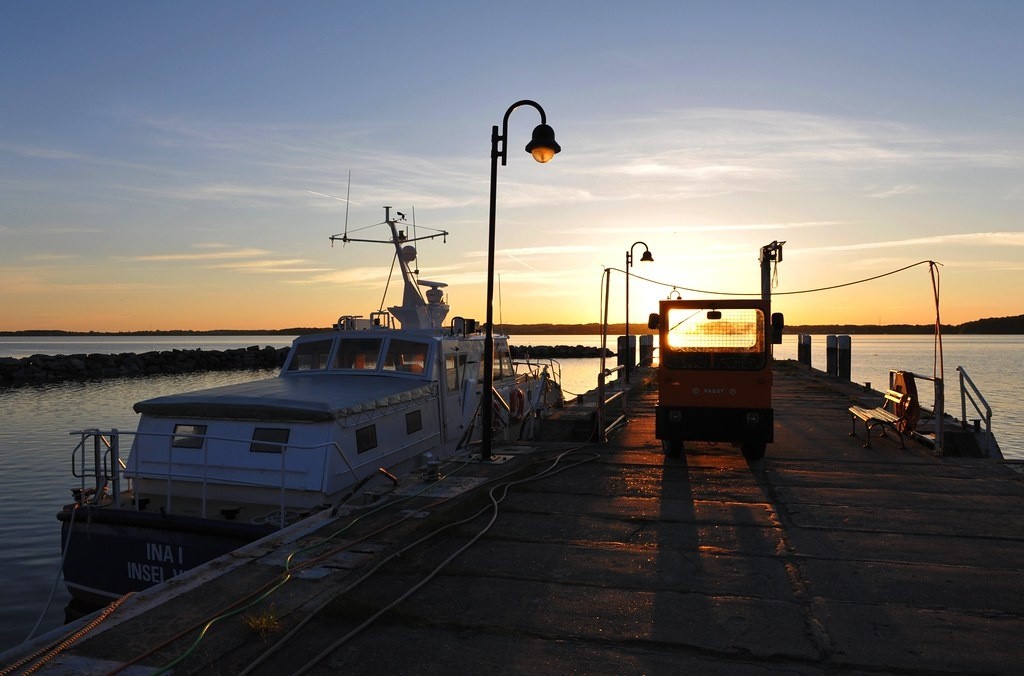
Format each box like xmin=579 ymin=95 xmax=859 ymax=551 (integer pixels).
xmin=848 ymin=388 xmax=915 ymax=449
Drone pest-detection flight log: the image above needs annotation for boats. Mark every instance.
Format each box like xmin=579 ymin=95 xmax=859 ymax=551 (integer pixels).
xmin=58 ymin=206 xmax=567 ymax=605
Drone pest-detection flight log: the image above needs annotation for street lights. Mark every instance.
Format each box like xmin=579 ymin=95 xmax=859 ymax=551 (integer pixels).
xmin=626 ymin=241 xmax=655 ymax=386
xmin=481 ymin=100 xmax=561 ymax=462
